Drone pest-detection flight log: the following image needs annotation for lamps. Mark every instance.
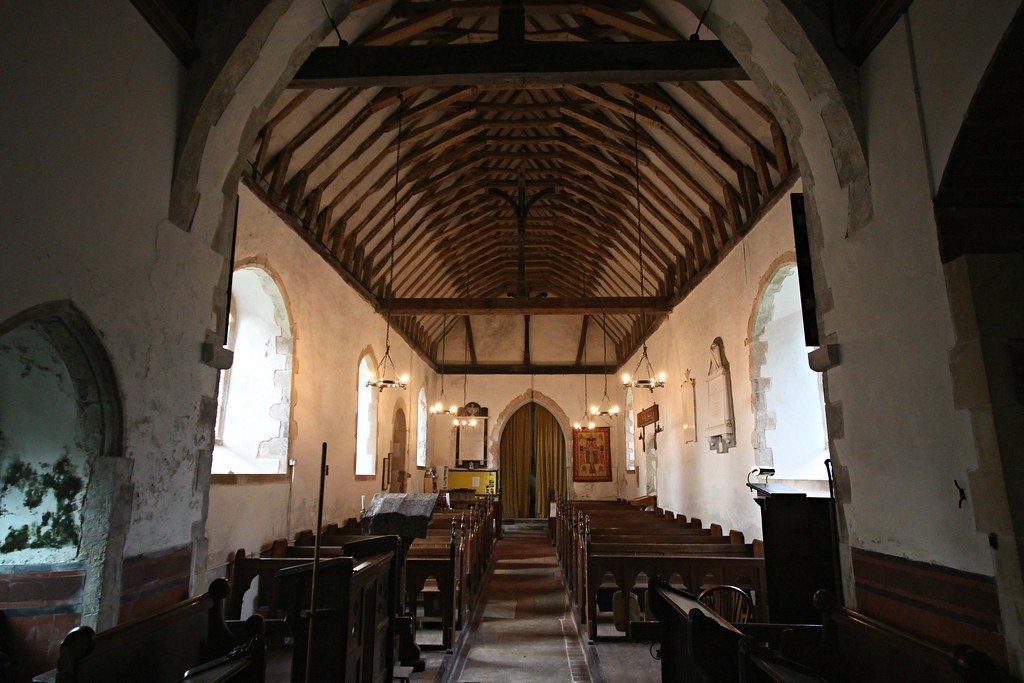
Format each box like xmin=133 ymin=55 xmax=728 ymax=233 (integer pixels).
xmin=429 ymin=314 xmax=458 ymax=417
xmin=574 ymin=269 xmax=597 ymax=430
xmin=590 ymin=313 xmax=619 ymax=420
xmin=453 ymin=267 xmax=477 ymax=428
xmin=365 ymin=90 xmax=410 ymax=392
xmin=621 ymin=93 xmax=666 ymax=393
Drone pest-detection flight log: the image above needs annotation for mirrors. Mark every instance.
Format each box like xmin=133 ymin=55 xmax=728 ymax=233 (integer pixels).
xmin=455 ymin=402 xmax=488 ymax=468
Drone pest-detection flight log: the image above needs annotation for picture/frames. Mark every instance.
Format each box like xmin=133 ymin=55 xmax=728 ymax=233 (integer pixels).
xmin=573 ymin=427 xmax=612 ymax=482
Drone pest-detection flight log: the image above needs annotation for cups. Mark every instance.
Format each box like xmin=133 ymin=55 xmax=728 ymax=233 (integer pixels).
xmin=469 ymin=462 xmax=473 ymax=470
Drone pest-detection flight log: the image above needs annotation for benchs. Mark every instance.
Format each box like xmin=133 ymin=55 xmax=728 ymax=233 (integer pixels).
xmin=34 ymin=488 xmax=503 ymax=683
xmin=557 ymin=497 xmax=766 ymax=641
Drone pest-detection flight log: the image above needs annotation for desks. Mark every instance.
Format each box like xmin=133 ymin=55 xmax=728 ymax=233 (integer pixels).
xmin=447 ymin=469 xmax=499 ymax=495
